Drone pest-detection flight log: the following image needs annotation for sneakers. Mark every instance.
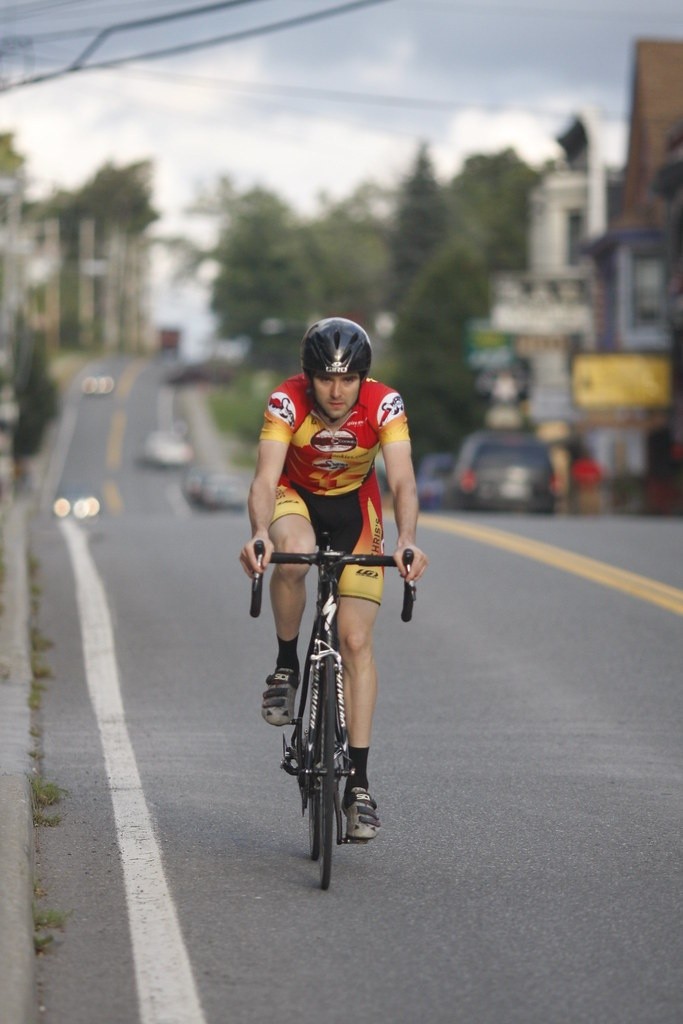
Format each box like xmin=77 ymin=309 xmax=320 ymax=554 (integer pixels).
xmin=341 ymin=786 xmax=381 ymax=839
xmin=261 ymin=668 xmax=301 ymax=726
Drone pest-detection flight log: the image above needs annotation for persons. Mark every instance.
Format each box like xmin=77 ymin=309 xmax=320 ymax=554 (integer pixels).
xmin=239 ymin=317 xmax=429 ymax=839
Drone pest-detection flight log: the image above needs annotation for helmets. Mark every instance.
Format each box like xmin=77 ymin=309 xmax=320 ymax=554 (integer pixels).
xmin=300 ymin=317 xmax=372 ymax=383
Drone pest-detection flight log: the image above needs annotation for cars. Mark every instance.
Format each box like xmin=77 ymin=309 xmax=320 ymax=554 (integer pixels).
xmin=140 ymin=429 xmax=192 ymax=468
xmin=413 ymin=451 xmax=453 ymax=510
xmin=187 ymin=467 xmax=249 ymax=512
xmin=447 ymin=429 xmax=554 ymax=514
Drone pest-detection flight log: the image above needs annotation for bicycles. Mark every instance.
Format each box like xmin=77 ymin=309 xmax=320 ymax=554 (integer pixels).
xmin=250 ymin=540 xmax=419 ymax=891
